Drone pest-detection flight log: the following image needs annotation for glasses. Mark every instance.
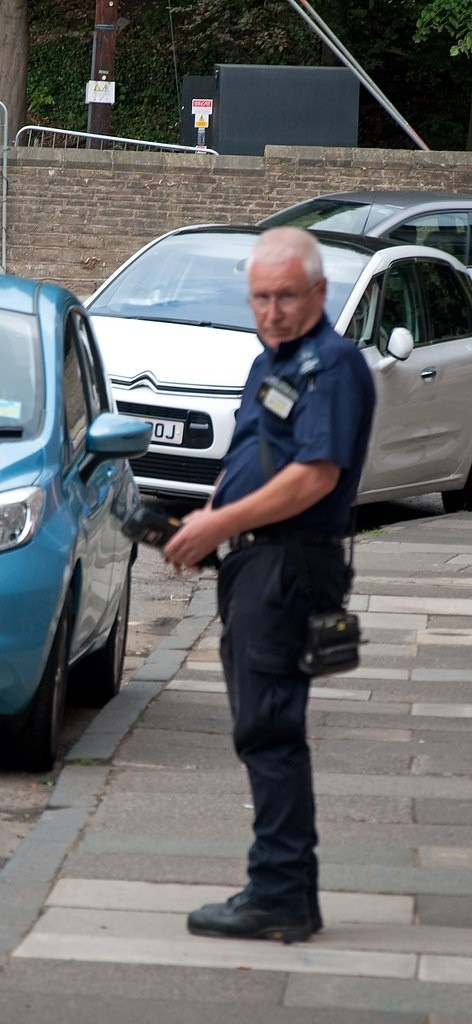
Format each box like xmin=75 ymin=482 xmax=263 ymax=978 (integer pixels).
xmin=246 ymin=285 xmax=318 ymax=313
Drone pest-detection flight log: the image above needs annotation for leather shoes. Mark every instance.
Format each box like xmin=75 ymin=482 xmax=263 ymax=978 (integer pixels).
xmin=187 ymin=892 xmax=308 ymax=942
xmin=200 ymin=892 xmax=322 ymax=931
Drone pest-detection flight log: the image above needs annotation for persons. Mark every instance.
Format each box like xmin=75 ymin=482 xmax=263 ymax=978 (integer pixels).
xmin=160 ymin=225 xmax=379 ymax=942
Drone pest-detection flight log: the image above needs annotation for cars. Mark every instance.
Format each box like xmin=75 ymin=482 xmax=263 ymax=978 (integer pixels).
xmin=0 ymin=274 xmax=153 ymax=771
xmin=72 ymin=223 xmax=472 ymax=501
xmin=255 ymin=191 xmax=472 ymax=275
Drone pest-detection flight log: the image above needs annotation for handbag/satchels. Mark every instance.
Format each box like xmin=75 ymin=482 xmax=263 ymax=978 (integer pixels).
xmin=299 ymin=614 xmax=360 ymax=675
